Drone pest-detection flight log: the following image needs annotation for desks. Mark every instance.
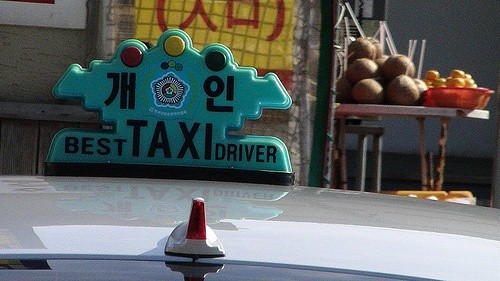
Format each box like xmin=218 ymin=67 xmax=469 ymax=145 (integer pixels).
xmin=333 ymin=102 xmax=491 ymax=192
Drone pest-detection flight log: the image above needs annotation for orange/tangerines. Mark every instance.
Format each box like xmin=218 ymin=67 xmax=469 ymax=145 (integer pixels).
xmin=423 ymin=69 xmax=478 ymax=89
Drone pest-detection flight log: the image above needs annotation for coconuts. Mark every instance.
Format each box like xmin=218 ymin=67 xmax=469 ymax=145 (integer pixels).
xmin=336 ymin=36 xmax=428 ymax=106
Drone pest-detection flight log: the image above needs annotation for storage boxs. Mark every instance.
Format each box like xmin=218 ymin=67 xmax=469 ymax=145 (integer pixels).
xmin=423 ymin=86 xmax=491 ymax=111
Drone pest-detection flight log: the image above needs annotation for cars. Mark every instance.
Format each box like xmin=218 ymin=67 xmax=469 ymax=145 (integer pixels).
xmin=0 ymin=30 xmax=500 ymax=280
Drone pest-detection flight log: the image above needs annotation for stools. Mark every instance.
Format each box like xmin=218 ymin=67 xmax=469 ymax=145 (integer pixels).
xmin=343 ymin=124 xmax=385 ymax=194
